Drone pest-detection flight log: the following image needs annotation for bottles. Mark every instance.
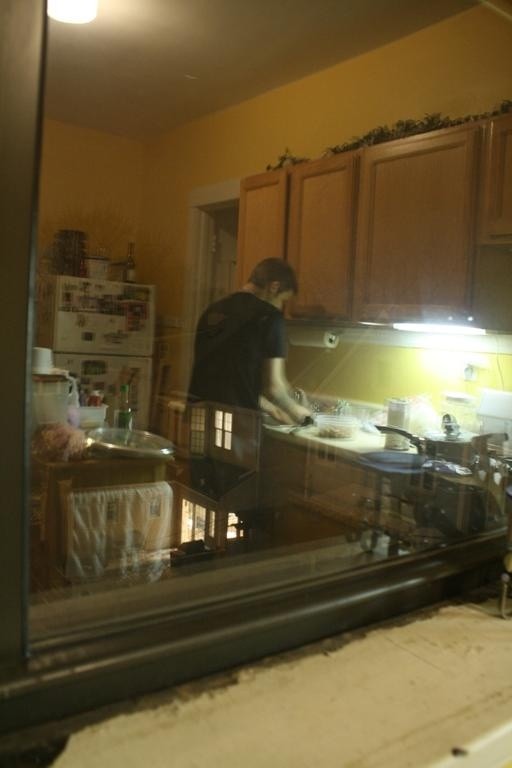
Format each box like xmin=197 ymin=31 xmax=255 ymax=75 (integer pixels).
xmin=114 ymin=383 xmax=132 ymax=428
xmin=123 ymin=240 xmax=136 ymax=283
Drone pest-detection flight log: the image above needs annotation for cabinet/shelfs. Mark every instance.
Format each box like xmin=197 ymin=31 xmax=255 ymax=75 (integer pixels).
xmin=237 ymin=151 xmax=356 ymax=328
xmin=360 ymin=111 xmax=510 ymax=336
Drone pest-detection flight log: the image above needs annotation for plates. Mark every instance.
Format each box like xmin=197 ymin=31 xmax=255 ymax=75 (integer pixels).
xmin=354 ymin=451 xmax=426 ymax=475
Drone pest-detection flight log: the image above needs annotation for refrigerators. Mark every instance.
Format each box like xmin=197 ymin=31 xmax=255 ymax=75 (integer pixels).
xmin=35 ymin=274 xmax=156 ymax=430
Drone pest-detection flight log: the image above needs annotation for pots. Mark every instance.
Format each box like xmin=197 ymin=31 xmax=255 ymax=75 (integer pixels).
xmin=374 ymin=423 xmax=477 ymax=466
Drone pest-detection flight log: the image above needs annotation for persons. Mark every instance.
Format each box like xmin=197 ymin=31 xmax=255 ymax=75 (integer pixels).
xmin=186 ymin=254 xmax=316 ymax=504
